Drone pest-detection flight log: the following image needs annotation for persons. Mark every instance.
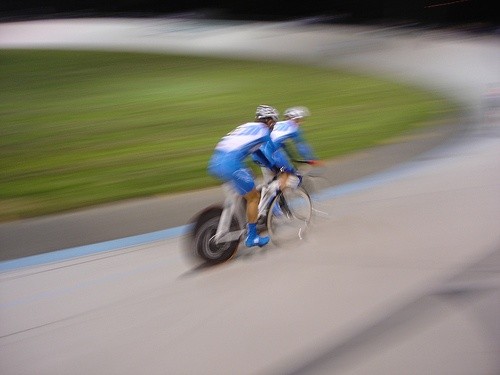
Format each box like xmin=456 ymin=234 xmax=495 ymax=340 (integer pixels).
xmin=250 ymin=106 xmax=317 ymax=224
xmin=207 ymin=104 xmax=295 ymax=247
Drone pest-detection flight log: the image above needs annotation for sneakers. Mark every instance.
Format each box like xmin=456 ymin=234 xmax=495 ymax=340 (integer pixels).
xmin=245 ymin=236 xmax=269 ymax=247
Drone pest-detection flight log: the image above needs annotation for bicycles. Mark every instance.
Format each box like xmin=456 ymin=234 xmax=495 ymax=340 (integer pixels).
xmin=182 ymin=168 xmax=313 ymax=265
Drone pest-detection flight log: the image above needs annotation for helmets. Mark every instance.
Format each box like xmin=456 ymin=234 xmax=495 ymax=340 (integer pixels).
xmin=254 ymin=105 xmax=280 ymax=122
xmin=284 ymin=107 xmax=304 ymax=119
